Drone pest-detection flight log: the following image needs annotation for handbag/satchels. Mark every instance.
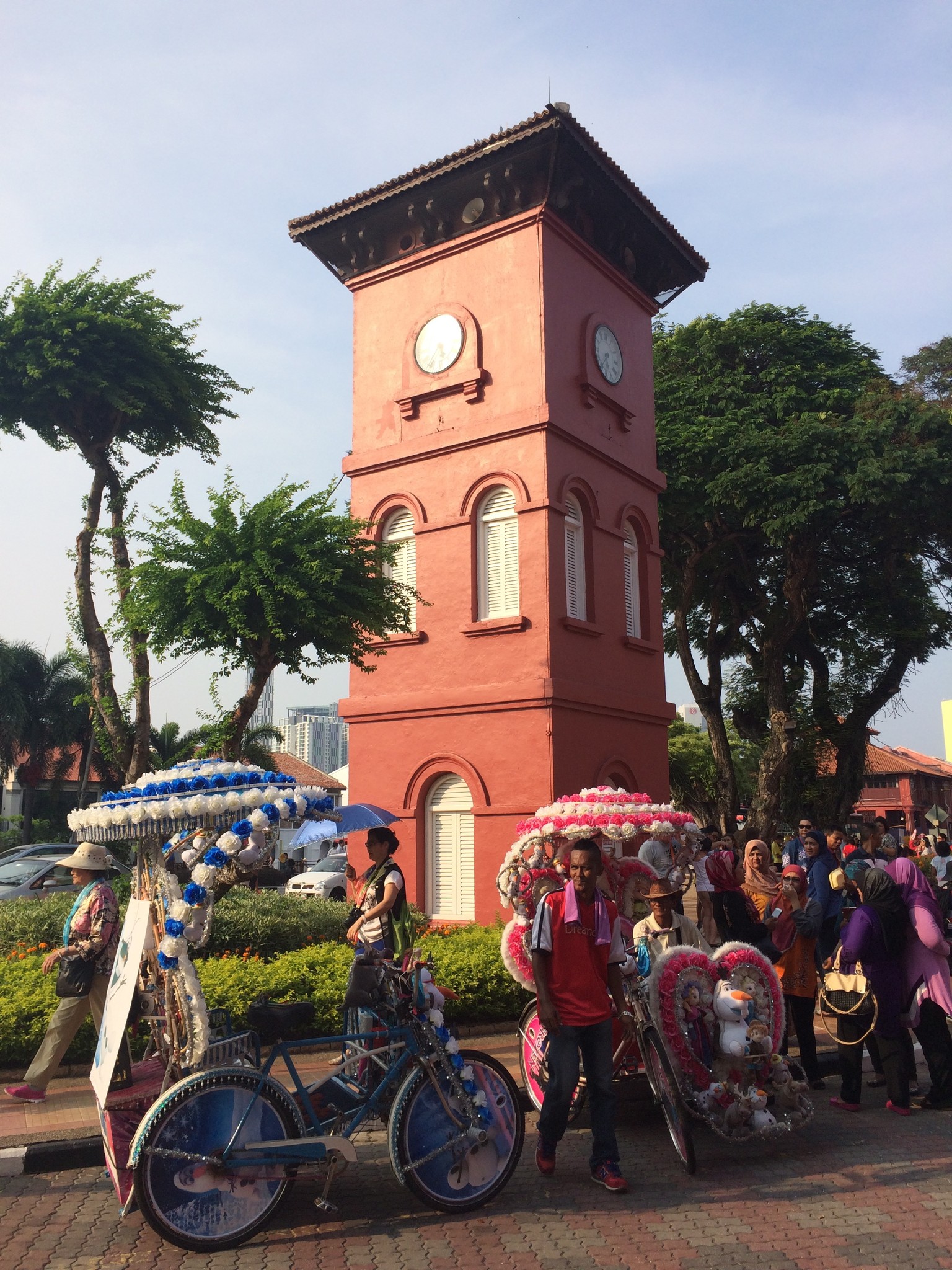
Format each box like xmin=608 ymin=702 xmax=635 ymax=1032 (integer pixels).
xmin=709 ymin=892 xmax=715 ymax=902
xmin=755 ymin=936 xmax=783 ymax=966
xmin=815 ymin=944 xmax=879 ymax=1045
xmin=55 ymin=958 xmax=97 ymax=997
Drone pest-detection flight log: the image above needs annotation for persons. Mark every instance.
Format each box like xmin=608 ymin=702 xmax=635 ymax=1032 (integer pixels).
xmin=278 ymin=853 xmax=289 ymax=873
xmin=530 ymin=838 xmax=637 ymax=1191
xmin=261 ymin=854 xmax=273 ymax=870
xmin=329 ymin=825 xmax=407 ymax=1066
xmin=631 ymin=878 xmax=714 ymax=979
xmin=686 ymin=815 xmax=952 ymax=1115
xmin=327 ymin=842 xmax=338 ymax=856
xmin=638 ymin=834 xmax=684 ymax=916
xmin=3 ymin=838 xmax=123 ymax=1103
xmin=336 ymin=839 xmax=345 ymax=854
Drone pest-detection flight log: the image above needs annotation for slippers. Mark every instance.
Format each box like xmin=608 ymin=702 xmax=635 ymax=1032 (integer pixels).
xmin=709 ymin=942 xmax=722 ymax=947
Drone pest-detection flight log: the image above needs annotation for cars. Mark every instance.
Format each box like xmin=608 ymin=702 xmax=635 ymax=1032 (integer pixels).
xmin=285 ymin=853 xmax=347 ymax=904
xmin=0 ymin=842 xmax=116 ymax=872
xmin=0 ymin=848 xmax=138 ymax=908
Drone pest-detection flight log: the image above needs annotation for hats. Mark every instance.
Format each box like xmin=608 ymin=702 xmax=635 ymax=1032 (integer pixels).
xmin=941 ymin=860 xmax=952 ymax=882
xmin=55 ymin=842 xmax=120 ymax=871
xmin=640 ymin=878 xmax=683 ymax=898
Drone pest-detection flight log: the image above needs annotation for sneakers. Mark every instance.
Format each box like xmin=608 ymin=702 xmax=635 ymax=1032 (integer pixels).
xmin=535 ymin=1130 xmax=558 ymax=1176
xmin=3 ymin=1084 xmax=47 ymax=1103
xmin=590 ymin=1156 xmax=628 ymax=1191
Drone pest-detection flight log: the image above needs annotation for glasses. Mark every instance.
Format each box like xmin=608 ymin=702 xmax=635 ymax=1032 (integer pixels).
xmin=798 ymin=825 xmax=814 ymax=830
xmin=783 ymin=877 xmax=800 ymax=883
xmin=648 ymin=898 xmax=673 ymax=903
xmin=938 ymin=838 xmax=942 ymax=839
xmin=364 ymin=841 xmax=381 ymax=847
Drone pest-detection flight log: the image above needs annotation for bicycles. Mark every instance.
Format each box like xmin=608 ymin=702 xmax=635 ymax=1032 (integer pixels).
xmin=126 ymin=952 xmax=524 ymax=1251
xmin=515 ymin=924 xmax=697 ymax=1170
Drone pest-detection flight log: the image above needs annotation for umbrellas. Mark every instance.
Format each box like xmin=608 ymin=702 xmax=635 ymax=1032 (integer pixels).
xmin=66 ymin=757 xmax=331 ymax=1067
xmin=287 ymin=803 xmax=403 ymax=878
xmin=517 ymin=785 xmax=699 ymax=839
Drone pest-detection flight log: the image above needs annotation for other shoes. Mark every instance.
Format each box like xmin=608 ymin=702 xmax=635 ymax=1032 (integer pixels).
xmin=886 ymin=1100 xmax=910 ymax=1115
xmin=812 ymin=1079 xmax=825 ymax=1089
xmin=919 ymin=1096 xmax=933 ymax=1107
xmin=866 ymin=1073 xmax=887 ymax=1086
xmin=327 ymin=1053 xmax=351 ymax=1065
xmin=829 ymin=1096 xmax=858 ymax=1111
xmin=908 ymin=1078 xmax=919 ymax=1094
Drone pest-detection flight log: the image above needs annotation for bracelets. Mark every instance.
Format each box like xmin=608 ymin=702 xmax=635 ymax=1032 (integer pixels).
xmin=361 ymin=914 xmax=367 ymax=925
xmin=617 ymin=1011 xmax=634 ymax=1020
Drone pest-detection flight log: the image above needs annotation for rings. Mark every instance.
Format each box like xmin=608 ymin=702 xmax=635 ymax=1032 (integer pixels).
xmin=628 ymin=1030 xmax=629 ymax=1032
